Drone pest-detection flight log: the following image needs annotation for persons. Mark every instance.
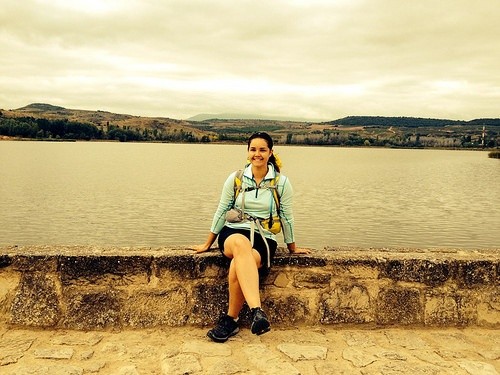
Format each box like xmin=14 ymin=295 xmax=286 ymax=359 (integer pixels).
xmin=184 ymin=131 xmax=312 ymax=343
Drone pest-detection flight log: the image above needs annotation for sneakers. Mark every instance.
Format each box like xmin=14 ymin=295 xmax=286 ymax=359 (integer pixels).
xmin=206 ymin=312 xmax=242 ymax=342
xmin=245 ymin=309 xmax=270 ymax=336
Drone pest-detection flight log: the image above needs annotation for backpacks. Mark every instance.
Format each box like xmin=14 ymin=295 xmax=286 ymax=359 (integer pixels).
xmin=227 ymin=154 xmax=283 ymax=235
